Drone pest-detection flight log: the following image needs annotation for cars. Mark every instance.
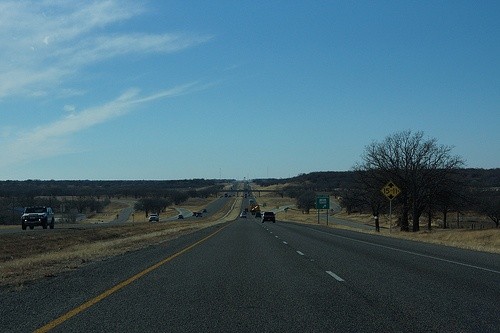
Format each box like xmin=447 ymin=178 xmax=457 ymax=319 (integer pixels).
xmin=249 ymin=199 xmax=261 ymax=218
xmin=148 ymin=214 xmax=161 ymax=222
xmin=244 ymin=208 xmax=248 ymax=212
xmin=261 ymin=211 xmax=276 ymax=224
xmin=239 ymin=212 xmax=247 ymax=218
xmin=178 ymin=215 xmax=184 ymax=220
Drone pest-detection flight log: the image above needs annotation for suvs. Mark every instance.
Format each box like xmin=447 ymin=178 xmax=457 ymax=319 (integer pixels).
xmin=20 ymin=205 xmax=55 ymax=230
